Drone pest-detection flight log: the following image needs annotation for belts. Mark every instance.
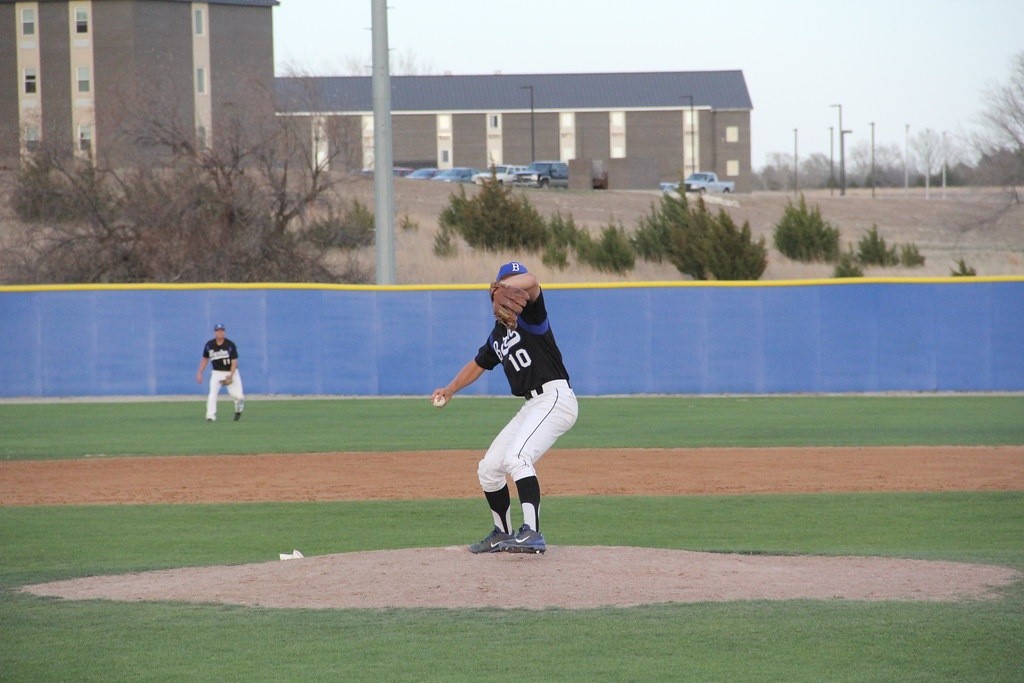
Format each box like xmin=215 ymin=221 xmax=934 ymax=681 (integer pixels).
xmin=525 ymin=379 xmax=570 ymax=400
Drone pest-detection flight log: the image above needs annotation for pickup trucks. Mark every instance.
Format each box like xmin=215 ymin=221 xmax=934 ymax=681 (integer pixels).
xmin=676 ymin=171 xmax=736 ymax=195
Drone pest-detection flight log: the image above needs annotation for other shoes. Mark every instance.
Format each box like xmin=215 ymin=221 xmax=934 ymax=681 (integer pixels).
xmin=234 ymin=412 xmax=241 ymax=421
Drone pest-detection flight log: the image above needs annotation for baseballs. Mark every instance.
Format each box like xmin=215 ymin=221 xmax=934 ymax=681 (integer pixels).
xmin=433 ymin=395 xmax=446 ymax=407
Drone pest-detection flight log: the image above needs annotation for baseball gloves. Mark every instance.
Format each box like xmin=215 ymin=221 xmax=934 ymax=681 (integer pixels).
xmin=488 ymin=281 xmax=531 ymax=331
xmin=219 ymin=376 xmax=233 ymax=385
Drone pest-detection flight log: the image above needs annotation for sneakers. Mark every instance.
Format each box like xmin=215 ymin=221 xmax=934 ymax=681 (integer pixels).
xmin=499 ymin=524 xmax=546 ymax=553
xmin=470 ymin=525 xmax=515 ymax=553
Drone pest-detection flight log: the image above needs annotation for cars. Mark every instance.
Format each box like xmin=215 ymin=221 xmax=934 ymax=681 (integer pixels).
xmin=469 ymin=164 xmax=529 ymax=188
xmin=392 ymin=167 xmax=414 ymax=178
xmin=430 ymin=166 xmax=481 ymax=185
xmin=404 ymin=167 xmax=440 ymax=179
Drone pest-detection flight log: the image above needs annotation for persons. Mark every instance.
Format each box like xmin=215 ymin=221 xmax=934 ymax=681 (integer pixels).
xmin=196 ymin=323 xmax=244 ymax=422
xmin=431 ymin=262 xmax=579 ymax=555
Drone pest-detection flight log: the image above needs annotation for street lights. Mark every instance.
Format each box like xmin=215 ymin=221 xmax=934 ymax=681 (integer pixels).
xmin=829 ymin=126 xmax=834 ymax=193
xmin=839 ymin=130 xmax=853 ymax=196
xmin=869 ymin=121 xmax=875 ymax=198
xmin=829 ymin=104 xmax=842 ymax=194
xmin=520 ymin=86 xmax=535 ymax=163
xmin=793 ymin=128 xmax=798 ymax=195
xmin=679 ymin=94 xmax=695 ymax=175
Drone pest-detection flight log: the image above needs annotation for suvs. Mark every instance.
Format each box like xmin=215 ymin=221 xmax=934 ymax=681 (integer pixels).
xmin=511 ymin=160 xmax=570 ymax=189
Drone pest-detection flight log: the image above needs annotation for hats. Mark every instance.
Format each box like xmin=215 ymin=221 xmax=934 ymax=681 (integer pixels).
xmin=496 ymin=262 xmax=528 ymax=282
xmin=215 ymin=324 xmax=225 ymax=330
